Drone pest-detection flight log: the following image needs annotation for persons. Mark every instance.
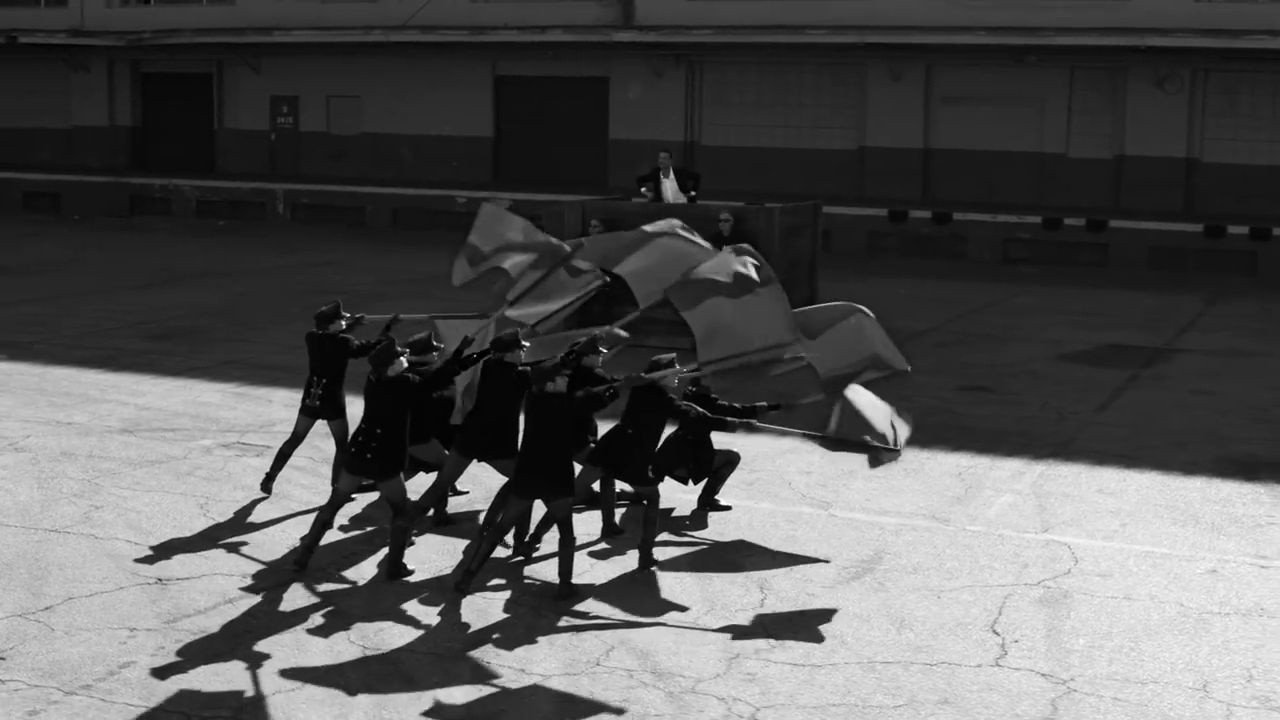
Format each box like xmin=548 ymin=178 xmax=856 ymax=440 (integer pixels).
xmin=708 ymin=208 xmax=738 ymax=251
xmin=636 ymin=149 xmax=702 ymax=203
xmin=587 ymin=217 xmax=605 ymax=235
xmin=260 ymin=300 xmax=783 ymax=599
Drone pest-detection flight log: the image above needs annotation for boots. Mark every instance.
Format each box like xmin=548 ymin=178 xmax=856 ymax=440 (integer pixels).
xmin=697 ymin=469 xmax=732 ymax=511
xmin=260 ymin=447 xmax=291 ymax=495
xmin=294 ymin=487 xmax=358 ymax=569
xmin=453 ymin=522 xmax=506 ymax=598
xmin=571 ymin=486 xmax=602 ymax=507
xmin=557 ymin=536 xmax=579 ymax=600
xmin=386 ymin=500 xmax=418 ymax=579
xmin=406 ymin=459 xmax=473 ymax=531
xmin=599 ymin=488 xmax=627 ymax=539
xmin=636 ymin=515 xmax=657 ymax=569
xmin=517 ymin=512 xmax=555 ymax=559
xmin=616 ymin=488 xmax=647 ymax=504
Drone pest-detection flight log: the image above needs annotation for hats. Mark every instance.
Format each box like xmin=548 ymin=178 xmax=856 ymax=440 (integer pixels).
xmin=488 ymin=327 xmax=531 ymax=352
xmin=529 ymin=360 xmax=573 ymax=377
xmin=647 ymin=352 xmax=685 ymax=376
xmin=313 ymin=299 xmax=351 ymax=322
xmin=568 ymin=337 xmax=608 ymax=356
xmin=367 ymin=337 xmax=410 ymax=369
xmin=401 ymin=330 xmax=445 ymax=357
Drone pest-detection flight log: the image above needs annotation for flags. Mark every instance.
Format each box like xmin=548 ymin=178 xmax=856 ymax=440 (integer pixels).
xmin=432 ymin=313 xmax=501 ymax=426
xmin=451 ymin=201 xmax=912 ymax=466
